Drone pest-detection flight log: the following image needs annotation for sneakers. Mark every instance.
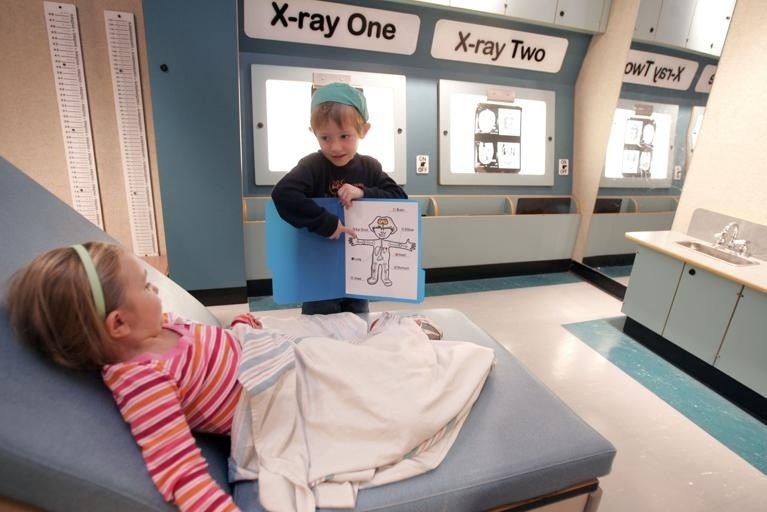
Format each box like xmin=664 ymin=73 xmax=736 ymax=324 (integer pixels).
xmin=369 ymin=316 xmax=444 ymax=342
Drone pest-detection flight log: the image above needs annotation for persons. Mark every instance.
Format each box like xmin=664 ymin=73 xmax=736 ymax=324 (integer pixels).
xmin=271 ymin=82 xmax=408 ymax=316
xmin=11 ymin=243 xmax=262 ymax=512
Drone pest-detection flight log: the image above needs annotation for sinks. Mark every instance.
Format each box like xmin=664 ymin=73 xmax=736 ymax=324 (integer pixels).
xmin=679 ymin=241 xmax=754 ymax=266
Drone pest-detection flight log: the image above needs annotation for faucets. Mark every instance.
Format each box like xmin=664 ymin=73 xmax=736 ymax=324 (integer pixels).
xmin=718 ymin=219 xmax=739 ymax=249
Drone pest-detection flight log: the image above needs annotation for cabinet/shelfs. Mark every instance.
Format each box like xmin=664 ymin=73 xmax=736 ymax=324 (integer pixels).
xmin=241 ymin=195 xmax=680 ymax=297
xmin=622 ymin=231 xmax=767 ymax=424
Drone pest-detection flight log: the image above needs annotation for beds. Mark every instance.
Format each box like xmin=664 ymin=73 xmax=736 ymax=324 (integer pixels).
xmin=0 ymin=161 xmax=617 ymax=512
